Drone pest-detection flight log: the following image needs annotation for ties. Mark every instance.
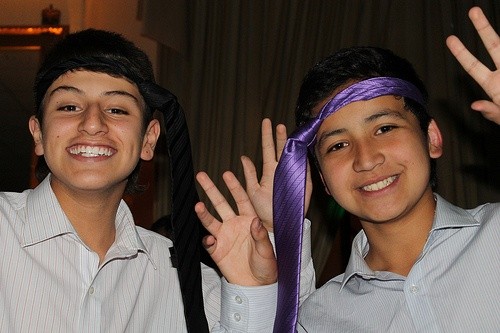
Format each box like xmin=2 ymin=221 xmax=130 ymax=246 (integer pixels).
xmin=273 ymin=76 xmax=422 ymax=333
xmin=34 ymin=55 xmax=210 ymax=333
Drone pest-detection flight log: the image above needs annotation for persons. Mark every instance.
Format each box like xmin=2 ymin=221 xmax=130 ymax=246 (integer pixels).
xmin=196 ymin=6 xmax=500 ymax=333
xmin=0 ymin=28 xmax=318 ymax=333
